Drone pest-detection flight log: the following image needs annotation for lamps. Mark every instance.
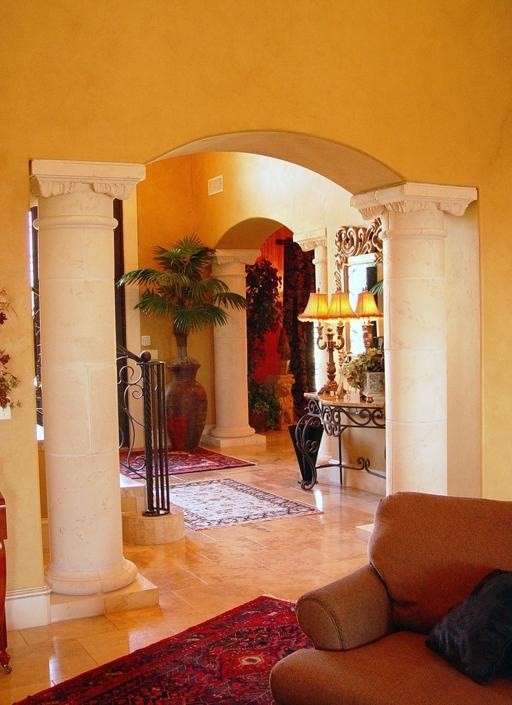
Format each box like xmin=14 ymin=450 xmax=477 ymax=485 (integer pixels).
xmin=354 ymin=285 xmax=383 ymax=351
xmin=295 ymin=287 xmax=358 ymax=399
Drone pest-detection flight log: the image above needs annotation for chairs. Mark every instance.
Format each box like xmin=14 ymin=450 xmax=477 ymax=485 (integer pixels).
xmin=269 ymin=490 xmax=512 ymax=705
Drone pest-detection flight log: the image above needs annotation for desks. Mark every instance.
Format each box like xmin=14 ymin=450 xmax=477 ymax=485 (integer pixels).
xmin=293 ymin=389 xmax=385 ymax=493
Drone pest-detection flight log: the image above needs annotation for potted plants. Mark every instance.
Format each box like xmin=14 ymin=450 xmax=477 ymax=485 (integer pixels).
xmin=248 ymin=378 xmax=282 ymax=431
xmin=346 ymin=347 xmax=385 ymax=396
xmin=114 ymin=232 xmax=247 ymax=452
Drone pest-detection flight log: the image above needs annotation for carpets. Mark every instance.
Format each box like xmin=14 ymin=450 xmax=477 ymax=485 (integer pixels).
xmin=16 ymin=594 xmax=318 ymax=705
xmin=151 ymin=477 xmax=325 ymax=531
xmin=115 ymin=444 xmax=257 ymax=479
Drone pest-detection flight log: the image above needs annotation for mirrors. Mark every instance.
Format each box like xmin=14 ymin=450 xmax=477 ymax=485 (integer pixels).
xmin=334 ymin=225 xmax=383 ymax=372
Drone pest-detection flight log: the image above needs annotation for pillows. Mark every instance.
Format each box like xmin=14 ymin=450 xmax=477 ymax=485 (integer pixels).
xmin=424 ymin=567 xmax=512 ymax=686
